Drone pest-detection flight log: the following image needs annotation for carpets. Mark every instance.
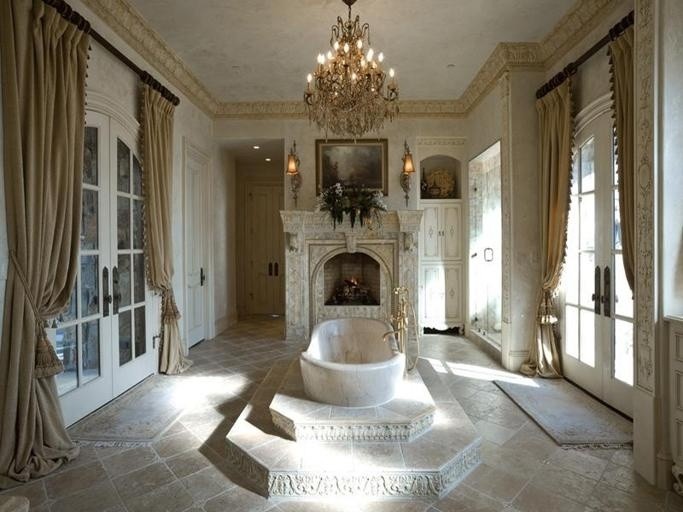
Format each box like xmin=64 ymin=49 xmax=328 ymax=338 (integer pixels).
xmin=492 ymin=376 xmax=634 ymax=450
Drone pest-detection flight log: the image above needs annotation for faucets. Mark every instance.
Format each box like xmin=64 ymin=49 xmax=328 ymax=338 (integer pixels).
xmin=382 ymin=328 xmax=401 ymax=343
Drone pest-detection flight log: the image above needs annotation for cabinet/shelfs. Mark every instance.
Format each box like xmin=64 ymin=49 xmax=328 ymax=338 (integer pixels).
xmin=417 ymin=203 xmax=464 ymax=336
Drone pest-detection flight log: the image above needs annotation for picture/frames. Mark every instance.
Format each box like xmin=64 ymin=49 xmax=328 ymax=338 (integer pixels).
xmin=314 ymin=138 xmax=389 ymax=197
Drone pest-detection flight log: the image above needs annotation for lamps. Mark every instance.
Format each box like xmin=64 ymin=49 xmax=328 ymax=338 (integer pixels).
xmin=301 ymin=0 xmax=400 ymax=145
xmin=285 ymin=139 xmax=302 ymax=208
xmin=399 ymin=138 xmax=416 ymax=208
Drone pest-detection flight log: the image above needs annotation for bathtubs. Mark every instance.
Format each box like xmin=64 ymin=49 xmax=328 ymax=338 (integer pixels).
xmin=298 ymin=317 xmax=404 ymax=406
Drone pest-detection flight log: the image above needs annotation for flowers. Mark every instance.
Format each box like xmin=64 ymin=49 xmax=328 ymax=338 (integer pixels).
xmin=315 ymin=176 xmax=388 ymax=232
